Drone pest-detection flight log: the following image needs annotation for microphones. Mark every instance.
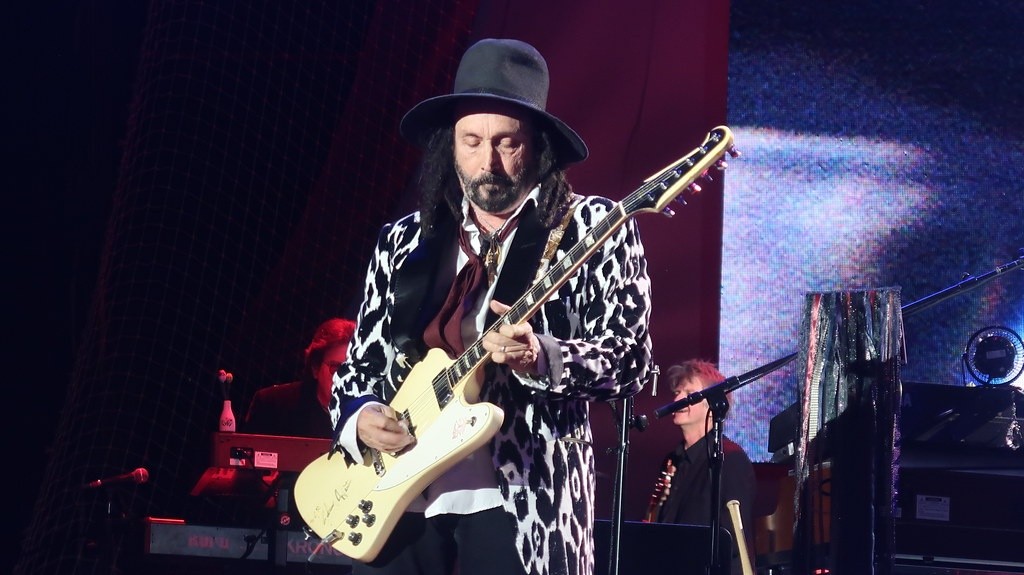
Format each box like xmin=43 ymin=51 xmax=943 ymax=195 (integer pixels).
xmin=637 ymin=415 xmax=649 ymax=432
xmin=88 ymin=467 xmax=150 ymax=488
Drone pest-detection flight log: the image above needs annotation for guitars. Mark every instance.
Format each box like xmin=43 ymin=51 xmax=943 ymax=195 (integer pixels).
xmin=291 ymin=124 xmax=742 ymax=562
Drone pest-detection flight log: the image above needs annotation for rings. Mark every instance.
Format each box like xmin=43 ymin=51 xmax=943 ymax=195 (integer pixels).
xmin=501 ymin=346 xmax=506 ymax=353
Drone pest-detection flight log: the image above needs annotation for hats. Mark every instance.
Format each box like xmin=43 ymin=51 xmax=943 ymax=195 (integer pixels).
xmin=400 ymin=38 xmax=589 ymax=163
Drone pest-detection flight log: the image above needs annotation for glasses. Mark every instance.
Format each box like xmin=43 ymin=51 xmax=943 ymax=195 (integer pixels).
xmin=322 ymin=360 xmax=340 ymax=374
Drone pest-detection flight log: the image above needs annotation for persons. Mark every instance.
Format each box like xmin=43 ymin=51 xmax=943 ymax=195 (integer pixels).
xmin=645 ymin=359 xmax=758 ymax=575
xmin=329 ymin=40 xmax=652 ymax=575
xmin=240 ymin=317 xmax=358 ymax=530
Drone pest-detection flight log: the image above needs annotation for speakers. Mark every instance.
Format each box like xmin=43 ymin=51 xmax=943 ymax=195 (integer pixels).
xmin=833 ymin=380 xmax=1024 ymax=575
